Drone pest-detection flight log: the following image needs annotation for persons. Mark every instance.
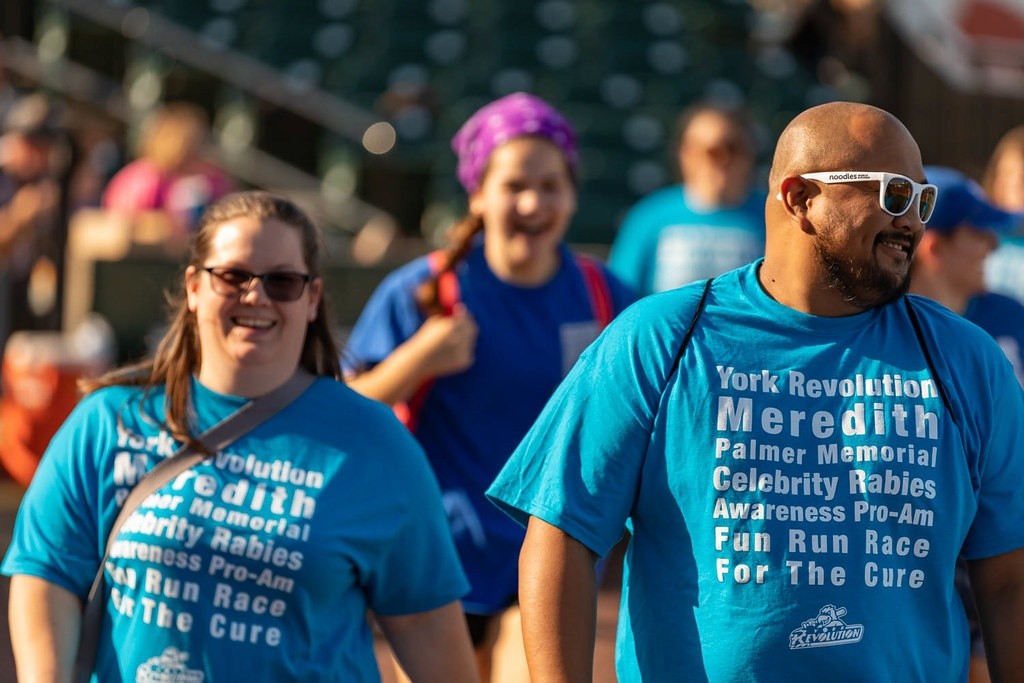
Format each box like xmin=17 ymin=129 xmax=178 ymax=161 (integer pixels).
xmin=0 ymin=67 xmax=245 ymax=332
xmin=337 ymin=92 xmax=644 ymax=683
xmin=784 ymin=0 xmax=910 ymax=120
xmin=604 ymin=107 xmax=770 ymax=297
xmin=905 ymin=122 xmax=1023 ymax=389
xmin=483 ymin=100 xmax=1024 ymax=683
xmin=315 ymin=64 xmax=464 ymax=268
xmin=0 ymin=189 xmax=482 ymax=683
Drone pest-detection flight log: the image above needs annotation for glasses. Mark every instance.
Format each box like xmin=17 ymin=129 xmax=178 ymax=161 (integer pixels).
xmin=777 ymin=171 xmax=939 ymax=224
xmin=200 ymin=266 xmax=315 ymax=302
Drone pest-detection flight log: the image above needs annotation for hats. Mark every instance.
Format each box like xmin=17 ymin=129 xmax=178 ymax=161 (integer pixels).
xmin=925 ymin=168 xmax=1022 ymax=235
